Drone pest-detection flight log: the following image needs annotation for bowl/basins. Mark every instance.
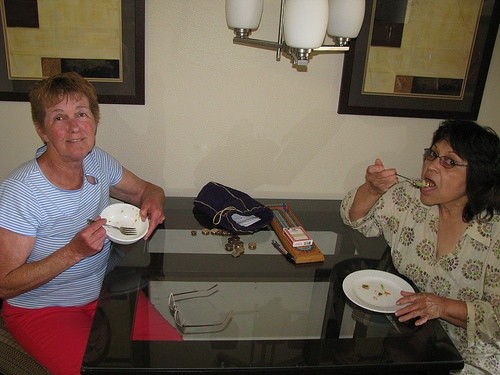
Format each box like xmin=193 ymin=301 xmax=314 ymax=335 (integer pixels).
xmin=99 ymin=203 xmax=150 ymax=245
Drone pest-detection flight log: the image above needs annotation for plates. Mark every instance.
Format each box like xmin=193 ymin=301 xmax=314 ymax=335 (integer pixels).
xmin=342 ymin=269 xmax=415 ymax=314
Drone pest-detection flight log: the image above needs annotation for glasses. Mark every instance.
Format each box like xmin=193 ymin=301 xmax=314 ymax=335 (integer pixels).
xmin=172 ymin=290 xmax=232 ymax=334
xmin=424 ymin=146 xmax=468 ymax=170
xmin=169 ymin=284 xmax=232 ymax=327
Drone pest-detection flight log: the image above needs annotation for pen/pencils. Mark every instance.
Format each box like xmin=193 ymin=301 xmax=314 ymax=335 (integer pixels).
xmin=271 ymin=239 xmax=295 ymax=262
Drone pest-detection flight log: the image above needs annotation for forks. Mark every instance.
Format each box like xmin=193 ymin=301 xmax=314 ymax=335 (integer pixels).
xmin=86 ymin=218 xmax=137 ymax=236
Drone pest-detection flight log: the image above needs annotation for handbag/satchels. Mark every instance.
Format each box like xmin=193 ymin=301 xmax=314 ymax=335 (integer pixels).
xmin=193 ymin=179 xmax=275 ymax=233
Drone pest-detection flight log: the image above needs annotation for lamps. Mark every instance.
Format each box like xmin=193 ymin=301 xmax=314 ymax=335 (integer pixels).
xmin=224 ymin=0 xmax=367 ymax=67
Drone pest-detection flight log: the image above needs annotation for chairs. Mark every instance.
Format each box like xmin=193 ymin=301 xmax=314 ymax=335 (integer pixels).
xmin=0 ymin=312 xmax=52 ymax=375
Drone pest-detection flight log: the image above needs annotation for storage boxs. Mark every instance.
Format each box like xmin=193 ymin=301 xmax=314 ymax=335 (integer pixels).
xmin=266 ymin=203 xmax=325 ymax=265
xmin=282 ymin=225 xmax=313 ymax=247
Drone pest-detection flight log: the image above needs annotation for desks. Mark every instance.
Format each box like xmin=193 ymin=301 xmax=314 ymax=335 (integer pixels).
xmin=79 ymin=196 xmax=465 ymax=375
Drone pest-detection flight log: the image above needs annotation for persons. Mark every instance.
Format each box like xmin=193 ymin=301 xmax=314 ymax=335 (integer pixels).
xmin=0 ymin=72 xmax=183 ymax=375
xmin=340 ymin=118 xmax=500 ymax=375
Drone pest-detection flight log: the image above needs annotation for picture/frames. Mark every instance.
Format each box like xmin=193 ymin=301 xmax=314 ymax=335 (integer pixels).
xmin=0 ymin=0 xmax=146 ymax=106
xmin=336 ymin=0 xmax=500 ymax=123
